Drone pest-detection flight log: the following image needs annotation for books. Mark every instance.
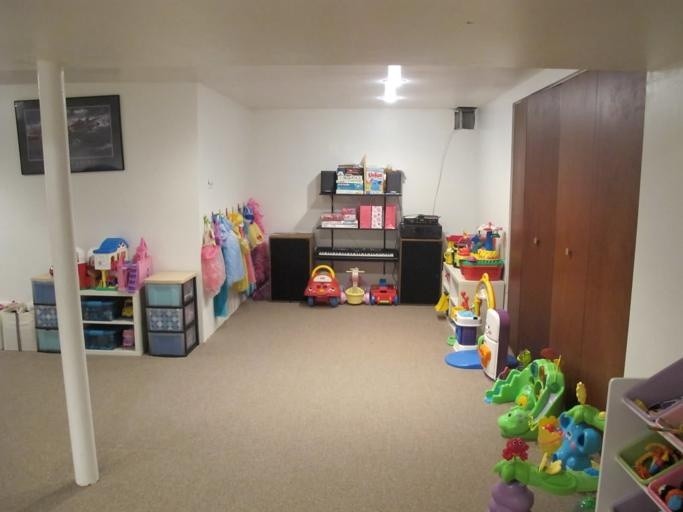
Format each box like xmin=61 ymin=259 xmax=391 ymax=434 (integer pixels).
xmin=365 ymin=168 xmax=384 ymax=194
xmin=319 ymin=164 xmax=364 ymax=229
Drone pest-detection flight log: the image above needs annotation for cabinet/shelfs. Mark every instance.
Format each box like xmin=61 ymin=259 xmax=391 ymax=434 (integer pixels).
xmin=594 ymin=377 xmax=683 ymax=512
xmin=440 ymin=260 xmax=504 ymax=336
xmin=78 ymin=289 xmax=146 ymax=358
xmin=317 ymin=191 xmax=402 ymax=274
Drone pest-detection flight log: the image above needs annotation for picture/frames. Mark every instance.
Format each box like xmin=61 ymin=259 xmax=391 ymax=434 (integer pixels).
xmin=14 ymin=95 xmax=124 ymax=174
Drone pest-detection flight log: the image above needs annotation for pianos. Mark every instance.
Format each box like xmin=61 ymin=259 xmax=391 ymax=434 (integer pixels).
xmin=314 ymin=246 xmax=400 ymax=263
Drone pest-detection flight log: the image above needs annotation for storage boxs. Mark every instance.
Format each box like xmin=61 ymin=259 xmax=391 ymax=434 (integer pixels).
xmin=617 ymin=355 xmax=683 ymax=512
xmin=81 ymin=301 xmax=118 ymax=322
xmin=0 ymin=278 xmax=62 ymax=356
xmin=143 ymin=272 xmax=198 ymax=357
xmin=84 ymin=327 xmax=121 ymax=350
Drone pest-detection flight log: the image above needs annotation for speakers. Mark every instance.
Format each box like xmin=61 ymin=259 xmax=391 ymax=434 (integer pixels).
xmin=397 ymin=231 xmax=443 ymax=306
xmin=386 ymin=170 xmax=402 ymax=193
xmin=454 ymin=107 xmax=478 ymax=130
xmin=320 ymin=171 xmax=335 ymax=193
xmin=268 ymin=231 xmax=316 ymax=301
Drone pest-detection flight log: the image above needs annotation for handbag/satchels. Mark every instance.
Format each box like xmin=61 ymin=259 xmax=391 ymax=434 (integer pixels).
xmin=202 ymin=243 xmax=226 ymax=299
